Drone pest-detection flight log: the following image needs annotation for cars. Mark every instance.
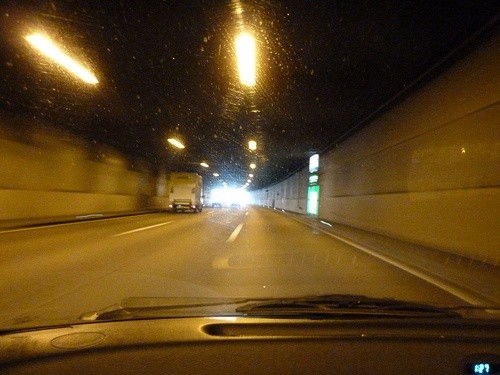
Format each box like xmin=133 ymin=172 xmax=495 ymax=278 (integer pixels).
xmin=212 ymin=199 xmax=241 ymax=206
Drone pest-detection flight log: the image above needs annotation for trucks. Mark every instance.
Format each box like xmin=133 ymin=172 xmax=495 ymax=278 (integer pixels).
xmin=168 ymin=172 xmax=206 ymax=213
